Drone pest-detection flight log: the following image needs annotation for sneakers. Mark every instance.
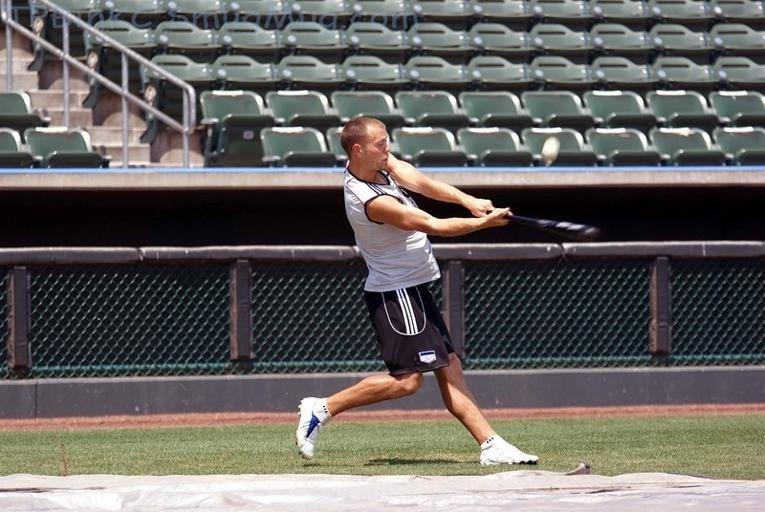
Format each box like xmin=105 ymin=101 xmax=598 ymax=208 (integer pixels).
xmin=293 ymin=395 xmax=333 ymax=458
xmin=477 ymin=430 xmax=540 ymax=468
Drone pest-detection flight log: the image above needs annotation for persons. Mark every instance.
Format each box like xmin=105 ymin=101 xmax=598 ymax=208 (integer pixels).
xmin=297 ymin=117 xmax=540 ymax=467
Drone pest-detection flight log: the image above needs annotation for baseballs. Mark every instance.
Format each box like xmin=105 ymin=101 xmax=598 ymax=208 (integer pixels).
xmin=542 ymin=137 xmax=560 ymax=161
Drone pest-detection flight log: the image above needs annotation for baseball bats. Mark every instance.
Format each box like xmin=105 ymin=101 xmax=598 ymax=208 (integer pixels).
xmin=486 ymin=209 xmax=598 ymax=236
